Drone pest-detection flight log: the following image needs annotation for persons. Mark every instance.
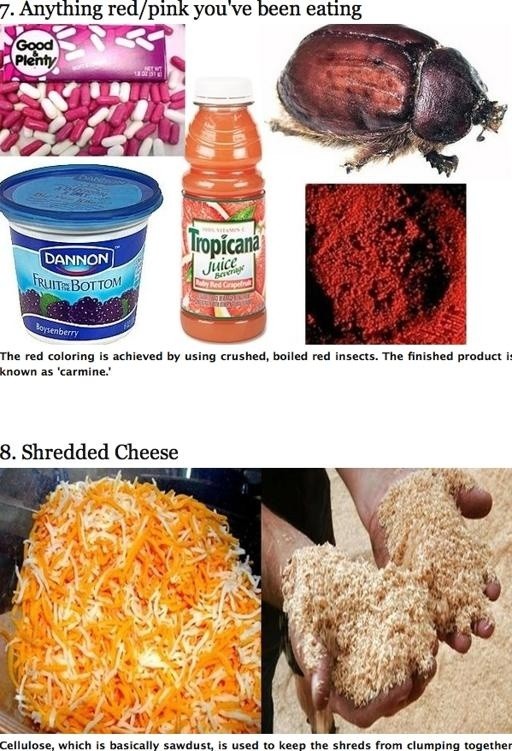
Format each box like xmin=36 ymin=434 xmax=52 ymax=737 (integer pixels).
xmin=257 ymin=468 xmax=501 ymax=730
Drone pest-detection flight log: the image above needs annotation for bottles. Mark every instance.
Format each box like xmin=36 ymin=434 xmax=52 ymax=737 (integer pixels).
xmin=181 ymin=78 xmax=269 ymax=344
